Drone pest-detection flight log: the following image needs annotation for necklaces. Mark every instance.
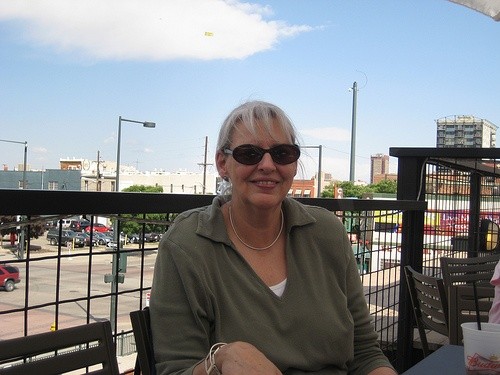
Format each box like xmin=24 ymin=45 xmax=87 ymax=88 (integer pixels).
xmin=229 ymin=206 xmax=285 ymax=250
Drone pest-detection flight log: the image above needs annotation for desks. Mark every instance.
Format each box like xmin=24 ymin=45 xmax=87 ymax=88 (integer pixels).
xmin=400 ymin=344 xmax=500 ymax=375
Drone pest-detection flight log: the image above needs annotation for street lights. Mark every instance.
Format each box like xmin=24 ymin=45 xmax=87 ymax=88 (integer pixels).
xmin=110 ymin=116 xmax=156 ymax=334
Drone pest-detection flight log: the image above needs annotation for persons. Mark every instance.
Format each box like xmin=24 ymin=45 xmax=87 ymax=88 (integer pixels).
xmin=149 ymin=101 xmax=400 ymax=375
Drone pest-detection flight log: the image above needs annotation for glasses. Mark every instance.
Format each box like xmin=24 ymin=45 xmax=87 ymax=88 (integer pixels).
xmin=222 ymin=142 xmax=301 ymax=165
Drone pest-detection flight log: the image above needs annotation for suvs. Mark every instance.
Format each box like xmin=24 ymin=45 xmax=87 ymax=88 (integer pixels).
xmin=46 ymin=229 xmax=87 ymax=248
xmin=56 ymin=219 xmax=91 ymax=233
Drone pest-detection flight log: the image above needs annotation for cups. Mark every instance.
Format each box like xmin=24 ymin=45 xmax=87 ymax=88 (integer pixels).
xmin=460 ymin=322 xmax=500 ymax=375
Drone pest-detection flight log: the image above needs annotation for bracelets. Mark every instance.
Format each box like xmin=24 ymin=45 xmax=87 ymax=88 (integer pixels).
xmin=204 ymin=343 xmax=227 ymax=375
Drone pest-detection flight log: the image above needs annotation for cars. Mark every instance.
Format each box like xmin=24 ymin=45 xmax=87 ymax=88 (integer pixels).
xmin=85 ymin=223 xmax=113 ymax=233
xmin=0 ymin=264 xmax=21 ymax=292
xmin=105 ymin=232 xmax=166 ymax=245
xmin=75 ymin=231 xmax=113 ymax=247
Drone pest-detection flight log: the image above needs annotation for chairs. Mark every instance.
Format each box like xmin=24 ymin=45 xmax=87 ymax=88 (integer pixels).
xmin=0 ymin=321 xmax=121 ymax=375
xmin=403 ymin=254 xmax=500 ymax=357
